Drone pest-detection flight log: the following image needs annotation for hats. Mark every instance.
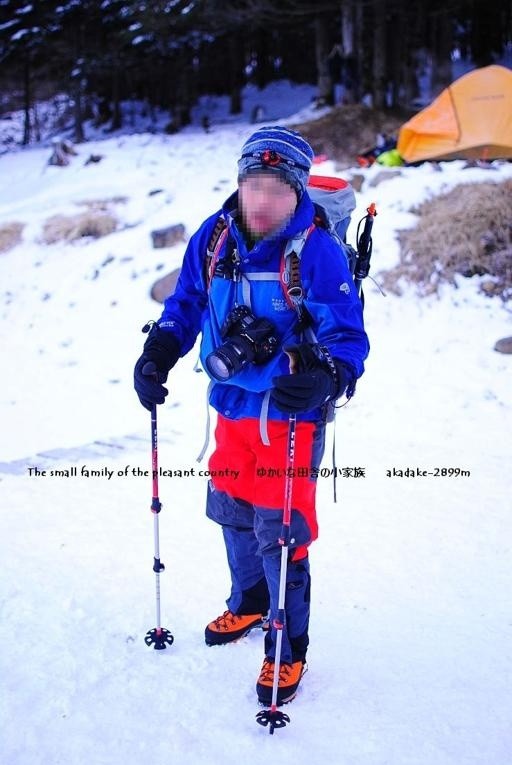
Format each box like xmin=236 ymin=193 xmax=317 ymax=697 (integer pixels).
xmin=238 ymin=126 xmax=313 ymax=202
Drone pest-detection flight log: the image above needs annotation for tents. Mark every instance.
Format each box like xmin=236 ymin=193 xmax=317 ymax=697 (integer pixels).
xmin=395 ymin=64 xmax=512 ymax=163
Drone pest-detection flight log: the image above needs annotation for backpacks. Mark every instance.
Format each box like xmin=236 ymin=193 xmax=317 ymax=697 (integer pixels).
xmin=205 ymin=175 xmax=356 ymax=327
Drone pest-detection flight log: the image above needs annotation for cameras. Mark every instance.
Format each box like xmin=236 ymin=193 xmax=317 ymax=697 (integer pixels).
xmin=206 ymin=305 xmax=281 ymax=382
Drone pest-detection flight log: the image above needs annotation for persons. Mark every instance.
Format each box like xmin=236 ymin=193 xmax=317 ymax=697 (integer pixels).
xmin=134 ymin=126 xmax=369 ymax=709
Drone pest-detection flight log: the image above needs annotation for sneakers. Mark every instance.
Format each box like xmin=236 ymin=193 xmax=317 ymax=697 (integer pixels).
xmin=205 ymin=610 xmax=268 ymax=642
xmin=257 ymin=659 xmax=306 ymax=702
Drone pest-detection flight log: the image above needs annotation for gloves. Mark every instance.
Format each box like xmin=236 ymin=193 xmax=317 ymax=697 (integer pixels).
xmin=269 ymin=343 xmax=347 ymax=413
xmin=134 ymin=332 xmax=180 ymax=411
